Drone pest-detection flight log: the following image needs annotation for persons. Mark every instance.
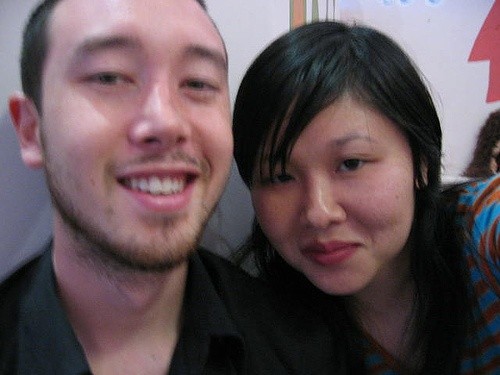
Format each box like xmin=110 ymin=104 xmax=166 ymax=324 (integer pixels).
xmin=231 ymin=21 xmax=499 ymax=374
xmin=1 ymin=1 xmax=336 ymax=375
xmin=460 ymin=112 xmax=500 ymax=178
